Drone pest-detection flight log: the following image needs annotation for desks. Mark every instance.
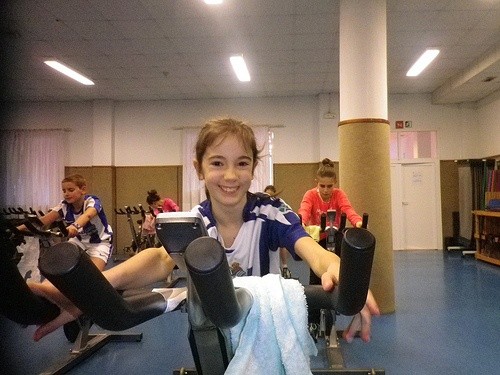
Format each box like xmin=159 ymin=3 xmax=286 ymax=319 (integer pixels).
xmin=471 ymin=210 xmax=500 ymax=266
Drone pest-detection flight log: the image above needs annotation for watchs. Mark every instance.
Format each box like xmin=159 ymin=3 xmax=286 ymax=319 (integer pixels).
xmin=72 ymin=222 xmax=80 ymax=228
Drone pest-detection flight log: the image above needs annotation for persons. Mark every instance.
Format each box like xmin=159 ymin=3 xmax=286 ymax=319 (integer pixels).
xmin=17 ymin=173 xmax=111 ymax=271
xmin=263 ymin=185 xmax=289 ymax=272
xmin=26 ymin=116 xmax=380 ymax=343
xmin=145 ymin=189 xmax=180 ymax=215
xmin=298 ymin=159 xmax=369 ymax=229
